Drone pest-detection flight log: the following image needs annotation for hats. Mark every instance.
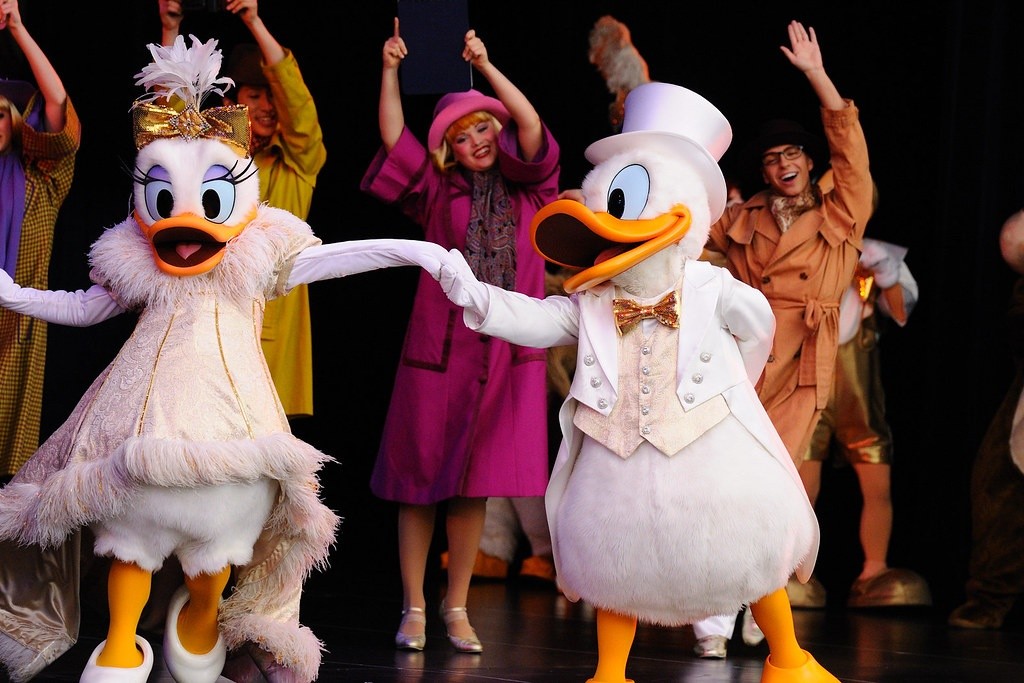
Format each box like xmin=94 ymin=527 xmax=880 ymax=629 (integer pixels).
xmin=756 ymin=121 xmax=822 ymax=174
xmin=427 ymin=88 xmax=512 ymax=155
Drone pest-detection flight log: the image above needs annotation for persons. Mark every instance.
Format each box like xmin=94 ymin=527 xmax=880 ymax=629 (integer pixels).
xmin=705 ymin=19 xmax=873 ymax=468
xmin=785 ymin=237 xmax=934 ymax=609
xmin=694 ymin=604 xmax=764 ymax=659
xmin=0 ymin=1 xmax=84 ymax=481
xmin=161 ymin=0 xmax=327 ymax=414
xmin=362 ymin=15 xmax=560 ymax=653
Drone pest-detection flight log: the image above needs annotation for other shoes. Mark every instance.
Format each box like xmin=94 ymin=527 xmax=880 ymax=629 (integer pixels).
xmin=690 ymin=634 xmax=731 ymax=659
xmin=742 ymin=605 xmax=765 ymax=647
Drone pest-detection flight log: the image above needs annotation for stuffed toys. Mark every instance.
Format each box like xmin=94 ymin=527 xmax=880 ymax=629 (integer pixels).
xmin=951 ymin=204 xmax=1024 ymax=627
xmin=0 ymin=33 xmax=487 ymax=683
xmin=442 ymin=495 xmax=555 ymax=578
xmin=442 ymin=83 xmax=842 ymax=683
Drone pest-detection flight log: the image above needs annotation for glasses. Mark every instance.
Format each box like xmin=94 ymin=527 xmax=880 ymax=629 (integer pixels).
xmin=760 ymin=145 xmax=804 ymax=166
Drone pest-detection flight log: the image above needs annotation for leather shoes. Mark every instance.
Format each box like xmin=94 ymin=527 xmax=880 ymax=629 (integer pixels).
xmin=437 ymin=598 xmax=485 ymax=654
xmin=395 ymin=606 xmax=428 ymax=652
